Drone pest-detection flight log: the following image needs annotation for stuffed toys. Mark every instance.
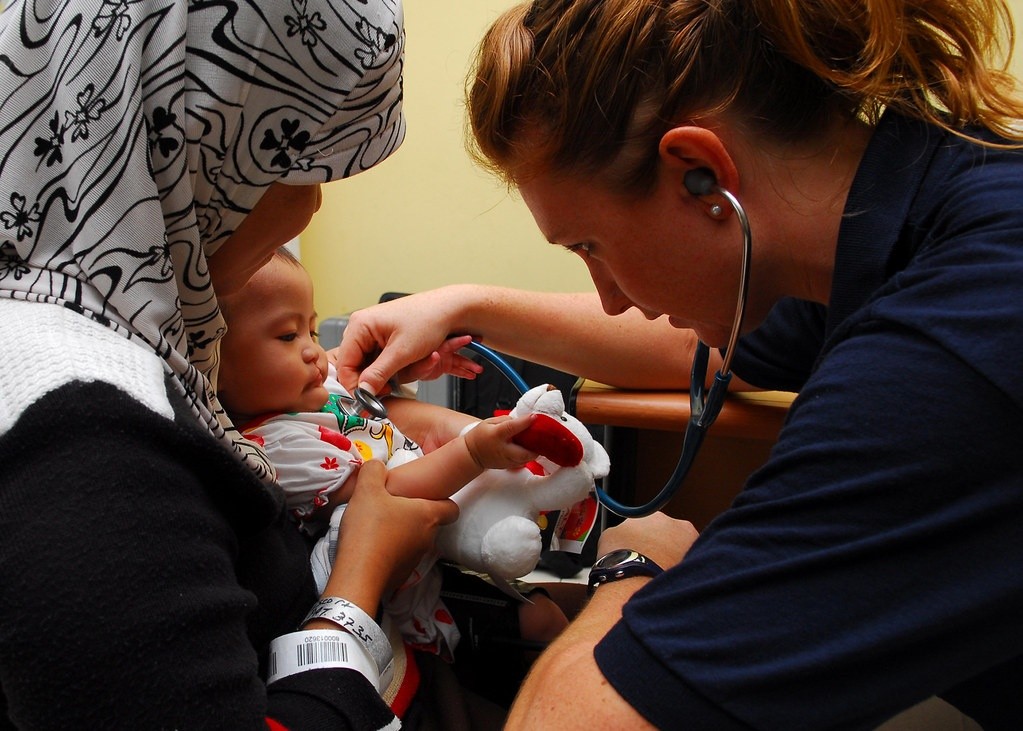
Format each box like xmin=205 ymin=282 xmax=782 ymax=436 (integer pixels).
xmin=386 ymin=383 xmax=611 ymax=581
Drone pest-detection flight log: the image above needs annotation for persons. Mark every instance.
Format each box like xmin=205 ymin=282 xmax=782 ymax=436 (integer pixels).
xmin=340 ymin=0 xmax=1023 ymax=731
xmin=219 ymin=244 xmax=570 ymax=731
xmin=0 ymin=0 xmax=462 ymax=731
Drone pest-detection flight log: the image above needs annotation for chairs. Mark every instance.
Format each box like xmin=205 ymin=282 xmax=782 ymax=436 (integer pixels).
xmin=315 ymin=317 xmax=463 ymax=414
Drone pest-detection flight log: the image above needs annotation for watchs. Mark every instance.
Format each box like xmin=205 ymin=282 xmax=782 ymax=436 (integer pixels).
xmin=587 ymin=549 xmax=664 ymax=601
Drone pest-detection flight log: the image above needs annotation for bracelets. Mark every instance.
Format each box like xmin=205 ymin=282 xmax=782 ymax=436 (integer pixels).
xmin=266 ymin=597 xmax=394 ymax=697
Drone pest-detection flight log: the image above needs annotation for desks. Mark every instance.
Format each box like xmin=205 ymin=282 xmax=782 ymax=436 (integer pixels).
xmin=573 ymin=377 xmax=801 ymax=542
